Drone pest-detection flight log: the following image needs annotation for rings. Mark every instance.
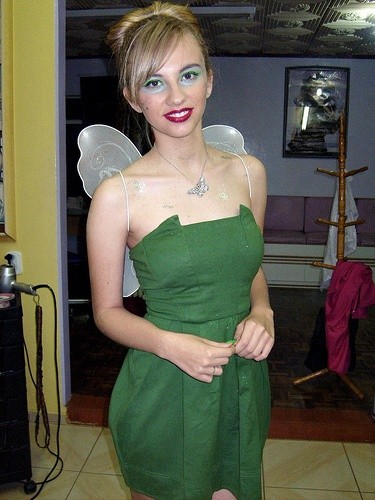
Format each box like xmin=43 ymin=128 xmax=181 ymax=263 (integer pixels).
xmin=210 ymin=366 xmax=216 ymax=376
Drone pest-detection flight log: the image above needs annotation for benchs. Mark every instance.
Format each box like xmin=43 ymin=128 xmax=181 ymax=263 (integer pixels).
xmin=260 ymin=195 xmax=375 ymax=290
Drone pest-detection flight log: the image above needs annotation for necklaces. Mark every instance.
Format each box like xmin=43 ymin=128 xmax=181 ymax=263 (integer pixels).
xmin=152 ymin=141 xmax=210 ymax=198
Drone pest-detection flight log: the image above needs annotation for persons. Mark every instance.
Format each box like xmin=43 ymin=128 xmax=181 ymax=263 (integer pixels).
xmin=86 ymin=2 xmax=277 ymax=500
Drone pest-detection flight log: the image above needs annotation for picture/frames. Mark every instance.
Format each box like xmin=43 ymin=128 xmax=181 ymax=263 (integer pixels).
xmin=282 ymin=66 xmax=351 ymax=160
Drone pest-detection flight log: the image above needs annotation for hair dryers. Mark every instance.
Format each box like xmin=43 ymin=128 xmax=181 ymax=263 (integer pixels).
xmin=0 ymin=265 xmax=36 ymax=296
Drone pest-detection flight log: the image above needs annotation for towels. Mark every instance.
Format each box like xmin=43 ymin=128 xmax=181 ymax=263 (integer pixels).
xmin=320 ymin=169 xmax=358 ymax=291
